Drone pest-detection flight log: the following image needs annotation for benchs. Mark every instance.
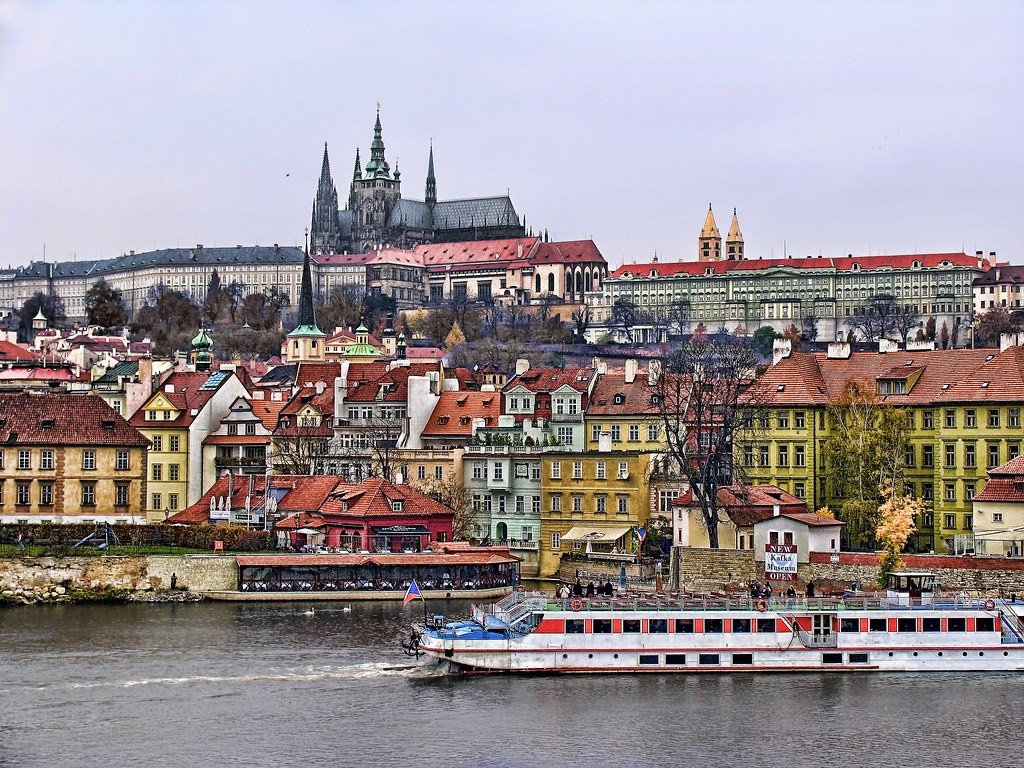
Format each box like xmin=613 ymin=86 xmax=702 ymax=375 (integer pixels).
xmin=580 ymin=591 xmax=882 ymax=608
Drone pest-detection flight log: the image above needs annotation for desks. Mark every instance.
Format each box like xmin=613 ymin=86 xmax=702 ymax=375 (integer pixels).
xmin=733 ymin=595 xmax=743 ymax=598
xmin=668 ymin=599 xmax=676 ymax=605
xmin=620 ymin=593 xmax=628 ymax=597
xmin=645 ymin=593 xmax=655 ymax=599
xmin=671 ymin=593 xmax=679 ymax=599
xmin=610 ymin=599 xmax=618 ymax=601
xmin=699 ymin=593 xmax=709 ymax=599
xmin=680 ymin=595 xmax=690 ymax=598
xmin=656 ymin=595 xmax=665 ymax=599
xmin=630 ymin=595 xmax=639 ymax=601
xmin=708 ymin=595 xmax=716 ymax=598
xmin=601 ymin=594 xmax=611 ymax=600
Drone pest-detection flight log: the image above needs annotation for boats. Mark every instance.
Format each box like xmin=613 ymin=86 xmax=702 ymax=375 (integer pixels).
xmin=198 ymin=551 xmax=520 ymax=602
xmin=401 ymin=573 xmax=1024 ymax=676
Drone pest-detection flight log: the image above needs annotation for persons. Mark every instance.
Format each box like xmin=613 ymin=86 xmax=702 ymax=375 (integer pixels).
xmin=748 ymin=578 xmax=919 ymax=599
xmin=277 ymin=542 xmax=448 ymax=554
xmin=555 ymin=579 xmax=613 ymax=599
xmin=17 ymin=530 xmax=24 ymax=549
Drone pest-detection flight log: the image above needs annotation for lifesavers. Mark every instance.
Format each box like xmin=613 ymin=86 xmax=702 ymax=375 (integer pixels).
xmin=570 ymin=600 xmax=583 ymax=611
xmin=757 ymin=600 xmax=768 ymax=612
xmin=984 ymin=599 xmax=996 ymax=610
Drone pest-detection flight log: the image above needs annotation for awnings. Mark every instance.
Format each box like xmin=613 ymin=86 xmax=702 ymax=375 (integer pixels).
xmin=560 ymin=527 xmax=630 ymax=544
xmin=371 ymin=525 xmax=431 ymax=535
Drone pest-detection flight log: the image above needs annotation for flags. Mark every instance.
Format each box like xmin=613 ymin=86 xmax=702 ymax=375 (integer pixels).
xmin=404 ymin=581 xmax=422 ymax=606
